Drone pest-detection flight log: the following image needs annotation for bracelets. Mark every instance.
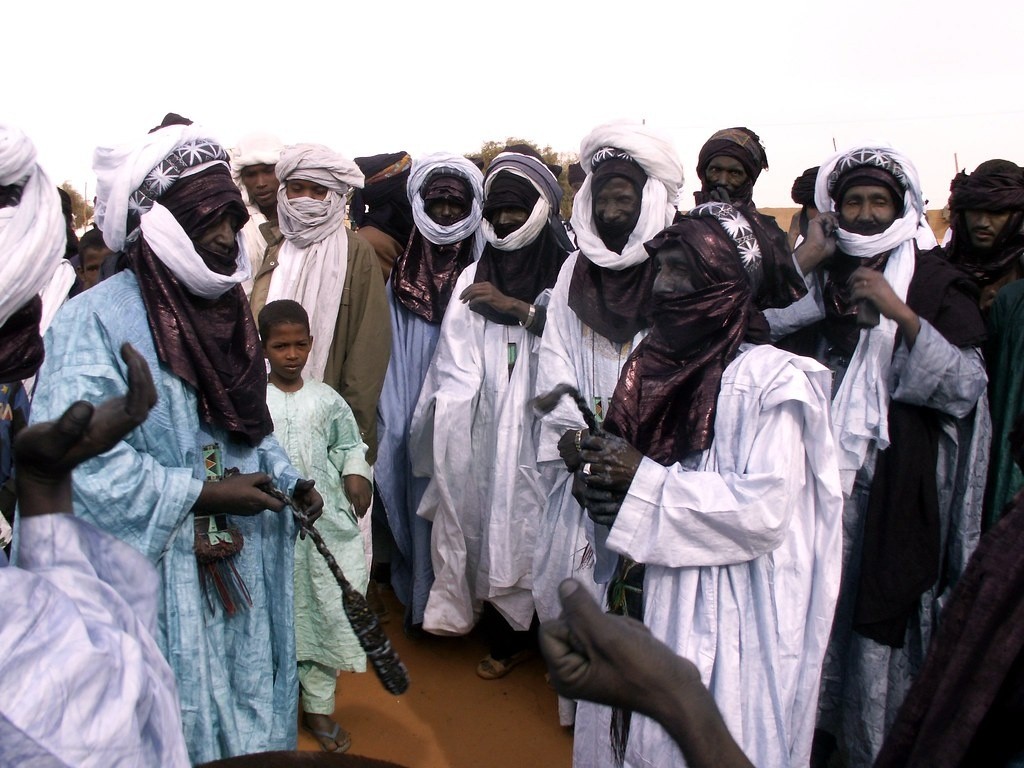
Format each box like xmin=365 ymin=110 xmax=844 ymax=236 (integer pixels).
xmin=519 ymin=303 xmax=535 ymax=328
xmin=574 ymin=430 xmax=583 ymax=452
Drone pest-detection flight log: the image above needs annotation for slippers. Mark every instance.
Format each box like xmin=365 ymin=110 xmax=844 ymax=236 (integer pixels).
xmin=299 ymin=712 xmax=353 ymax=753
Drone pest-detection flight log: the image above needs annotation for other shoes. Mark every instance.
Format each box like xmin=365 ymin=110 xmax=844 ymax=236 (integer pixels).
xmin=365 ymin=577 xmax=392 ymax=624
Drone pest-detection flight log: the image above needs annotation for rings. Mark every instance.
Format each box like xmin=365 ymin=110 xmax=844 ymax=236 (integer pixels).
xmin=583 ymin=463 xmax=592 ymax=477
xmin=863 ymin=280 xmax=868 ymax=288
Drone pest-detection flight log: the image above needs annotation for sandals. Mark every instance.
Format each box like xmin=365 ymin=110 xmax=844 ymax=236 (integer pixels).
xmin=544 ymin=661 xmax=557 ymax=691
xmin=477 ymin=649 xmax=526 ymax=680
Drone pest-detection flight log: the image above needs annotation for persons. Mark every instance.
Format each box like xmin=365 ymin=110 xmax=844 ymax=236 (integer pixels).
xmin=371 ymin=121 xmax=1024 ymax=766
xmin=0 ymin=106 xmax=408 ymax=768
